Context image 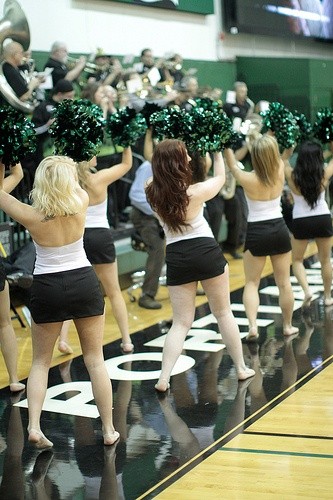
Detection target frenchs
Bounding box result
[0,0,41,113]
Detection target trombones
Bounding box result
[117,79,210,97]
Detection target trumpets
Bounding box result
[67,57,107,75]
[151,59,183,74]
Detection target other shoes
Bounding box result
[222,243,240,259]
[138,295,160,309]
[5,272,33,288]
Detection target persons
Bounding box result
[0,39,333,447]
[145,138,255,391]
[0,308,333,500]
